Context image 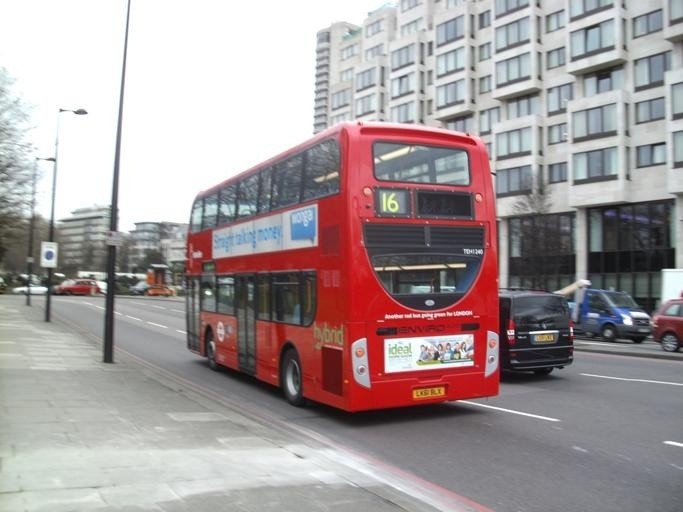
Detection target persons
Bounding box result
[420,342,467,362]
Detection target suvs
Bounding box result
[498,286,575,377]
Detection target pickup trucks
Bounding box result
[562,288,652,342]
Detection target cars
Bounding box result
[138,284,173,296]
[652,299,683,352]
[12,277,101,296]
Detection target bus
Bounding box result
[183,120,503,413]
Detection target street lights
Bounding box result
[44,107,88,323]
[24,156,56,306]
